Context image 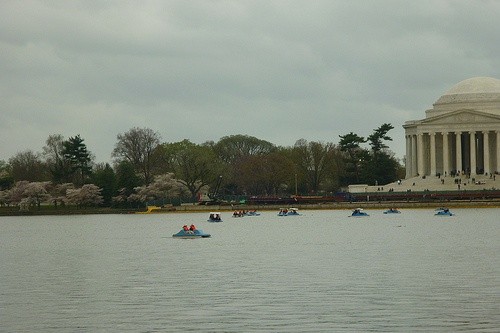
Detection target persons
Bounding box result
[254,209,256,212]
[239,210,246,215]
[183,225,189,231]
[281,209,288,213]
[424,189,429,191]
[398,179,401,185]
[378,187,383,190]
[215,214,220,220]
[485,171,498,181]
[444,208,449,213]
[389,188,393,192]
[210,214,214,219]
[438,169,480,190]
[352,210,357,215]
[234,210,237,215]
[190,224,196,231]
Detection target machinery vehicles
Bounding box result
[198,175,223,205]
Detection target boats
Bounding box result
[232,211,261,218]
[172,229,211,238]
[352,209,367,216]
[383,209,401,214]
[208,212,223,222]
[277,208,299,216]
[435,207,452,216]
